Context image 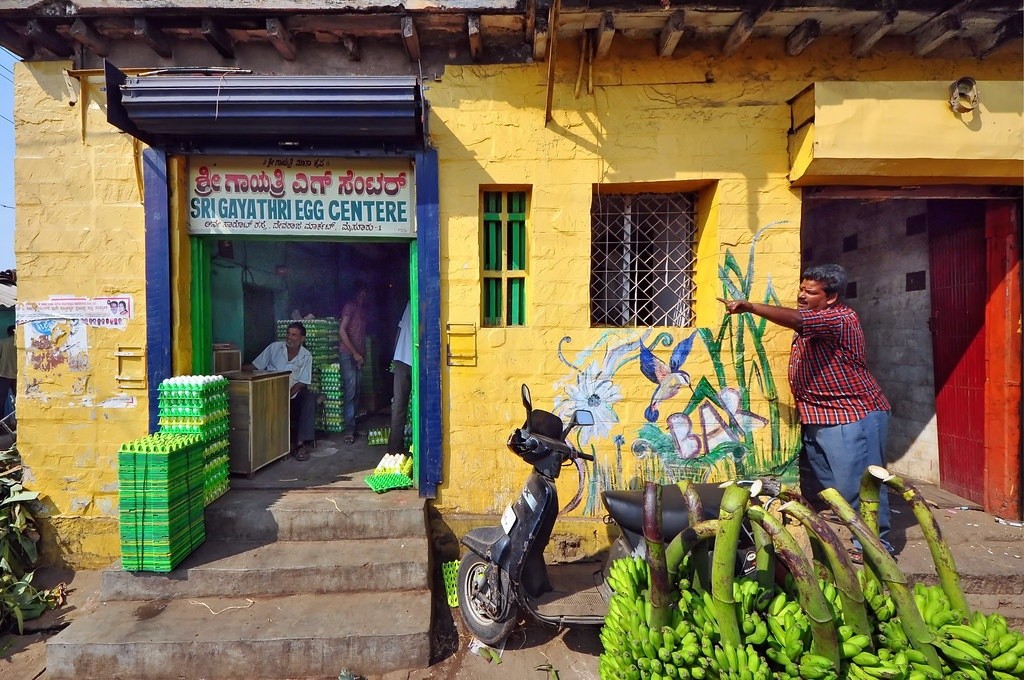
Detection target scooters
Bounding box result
[453,382,793,648]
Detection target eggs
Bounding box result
[162,374,227,501]
[369,431,381,436]
[377,452,404,470]
[277,319,345,431]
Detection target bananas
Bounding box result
[600,552,1024,680]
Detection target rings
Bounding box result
[733,302,735,305]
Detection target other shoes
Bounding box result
[818,510,845,525]
[343,431,355,445]
[296,447,309,461]
[847,548,865,564]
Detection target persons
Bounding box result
[338,280,370,445]
[716,264,896,564]
[110,301,128,315]
[387,300,411,457]
[0,325,16,427]
[242,322,317,461]
